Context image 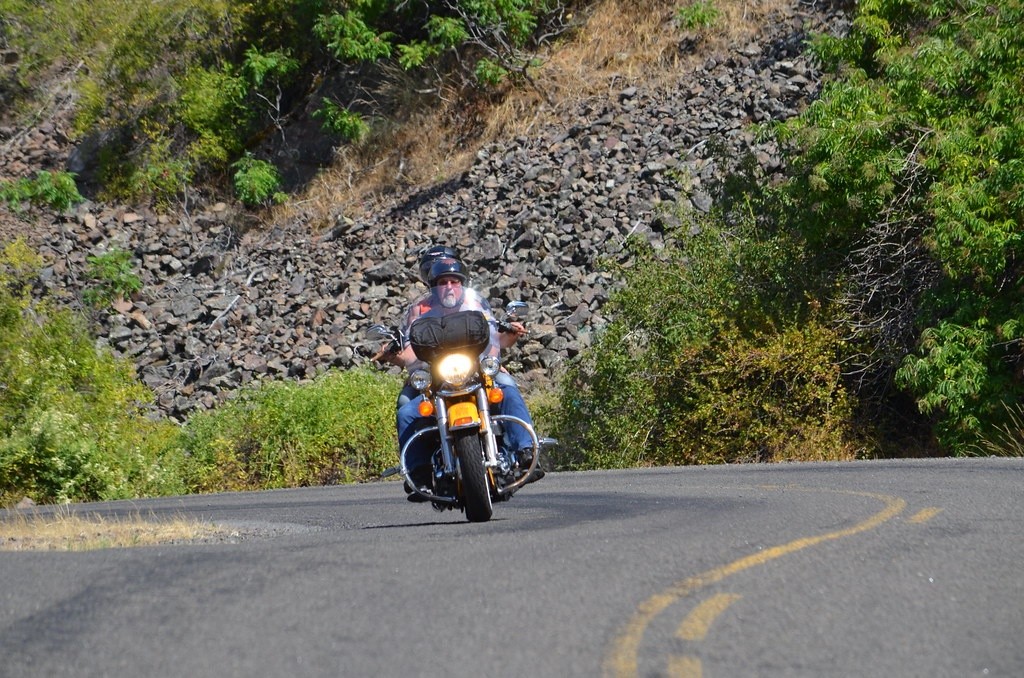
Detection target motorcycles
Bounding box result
[366,300,560,522]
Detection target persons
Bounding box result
[369,244,545,503]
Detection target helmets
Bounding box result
[427,258,470,287]
[419,246,462,287]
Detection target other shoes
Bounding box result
[411,475,427,489]
[519,450,545,481]
